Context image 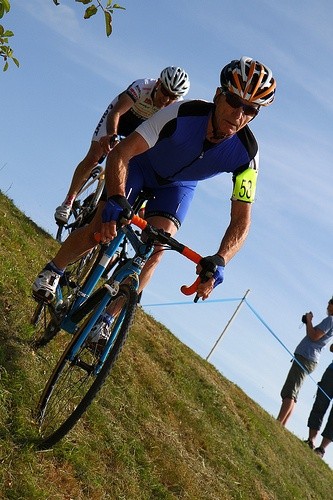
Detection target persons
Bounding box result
[276,298,333,427]
[30,57,277,349]
[54,66,191,223]
[304,343,333,458]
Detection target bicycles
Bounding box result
[54,133,121,283]
[29,188,221,451]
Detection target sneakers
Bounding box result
[89,323,111,350]
[54,205,70,223]
[32,268,61,301]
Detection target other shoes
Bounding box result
[304,439,313,448]
[314,447,325,457]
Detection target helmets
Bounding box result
[160,66,190,96]
[220,56,275,106]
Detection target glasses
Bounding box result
[161,83,178,100]
[222,87,259,116]
